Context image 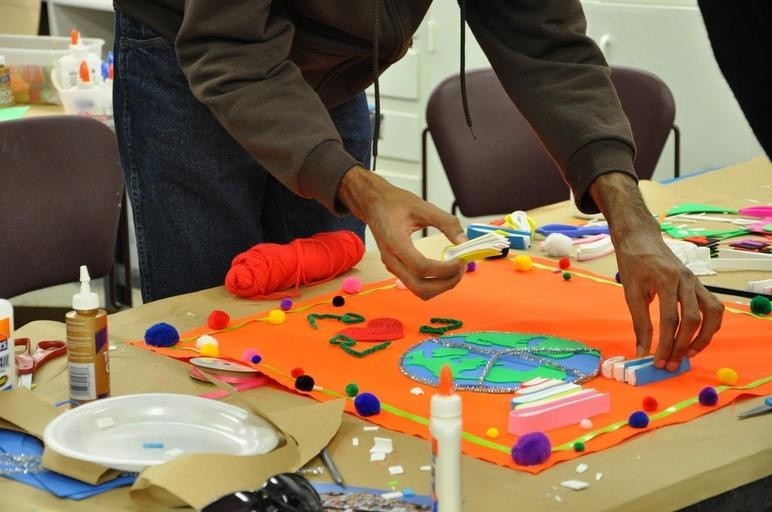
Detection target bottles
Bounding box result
[0,29,114,118]
[58,264,113,403]
[427,365,464,512]
[0,294,15,392]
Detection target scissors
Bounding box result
[14,338,67,388]
[736,396,772,418]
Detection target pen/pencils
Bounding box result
[320,449,344,485]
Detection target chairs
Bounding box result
[422,64,681,219]
[2,117,124,329]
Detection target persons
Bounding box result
[110,1,725,371]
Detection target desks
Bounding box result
[1,149,770,511]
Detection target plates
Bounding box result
[46,391,283,472]
